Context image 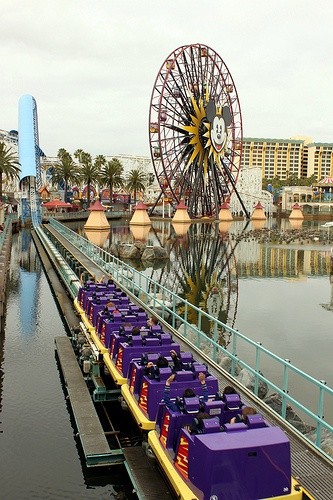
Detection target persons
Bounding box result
[231,209,270,217]
[0,204,18,235]
[43,206,176,216]
[83,272,258,437]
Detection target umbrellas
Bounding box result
[42,196,73,213]
